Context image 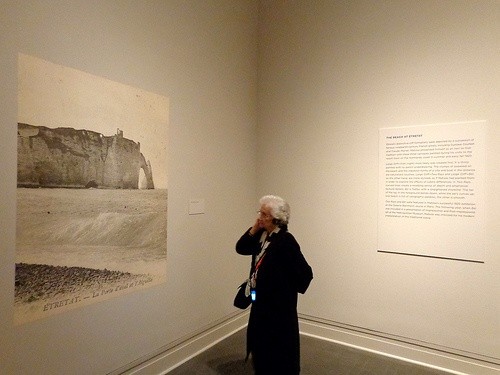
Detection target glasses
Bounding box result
[256,211,271,218]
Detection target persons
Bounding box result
[235,194,313,375]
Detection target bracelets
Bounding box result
[249,231,254,235]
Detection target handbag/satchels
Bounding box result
[233,282,252,309]
